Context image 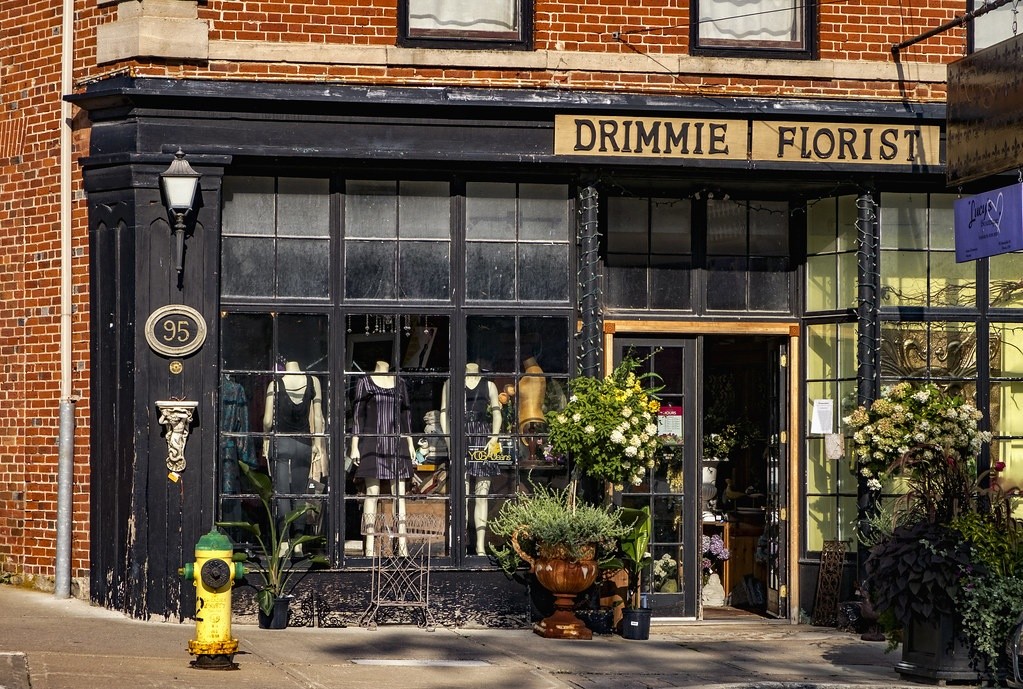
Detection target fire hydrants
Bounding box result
[177,525,250,671]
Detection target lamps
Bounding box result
[158,146,203,275]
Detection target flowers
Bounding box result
[654,553,677,587]
[702,534,730,585]
[543,342,670,488]
[666,425,739,529]
[841,384,1023,689]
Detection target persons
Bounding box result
[350,361,417,560]
[516,358,548,443]
[439,363,506,558]
[219,373,256,515]
[263,360,323,558]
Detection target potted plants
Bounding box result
[213,459,332,631]
[486,486,641,640]
[599,503,651,639]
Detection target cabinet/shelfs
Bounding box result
[675,519,730,610]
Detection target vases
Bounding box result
[660,579,676,592]
[900,593,979,681]
[702,457,731,521]
